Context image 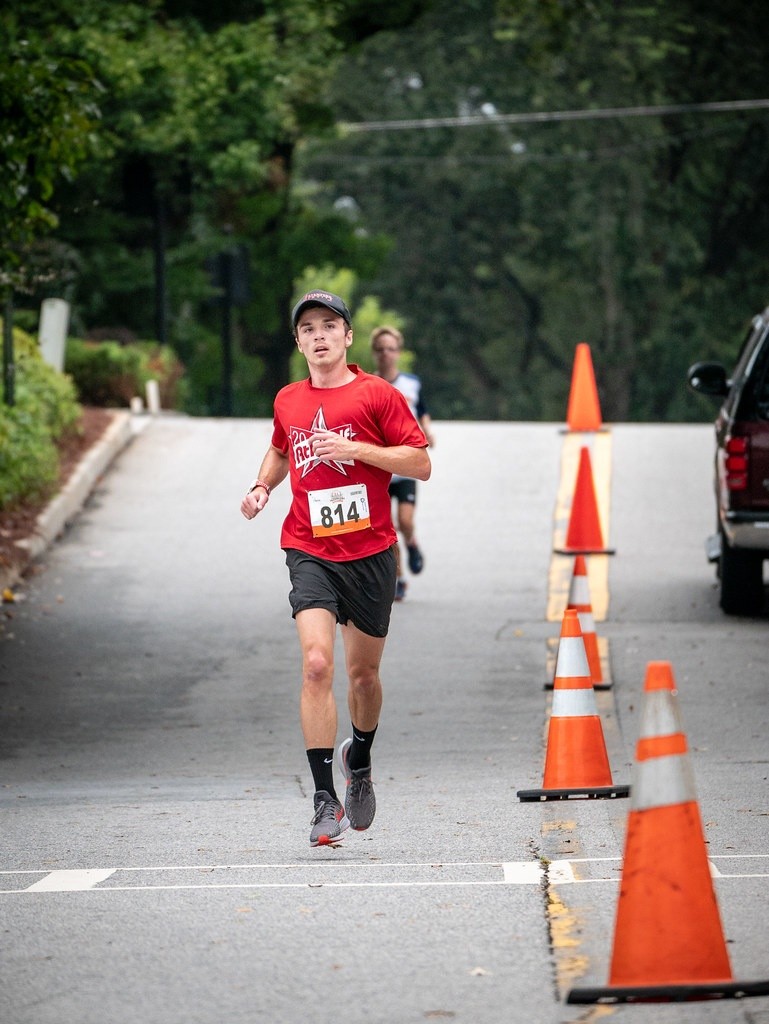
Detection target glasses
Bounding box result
[375,347,402,356]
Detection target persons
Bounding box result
[366,325,436,601]
[241,289,433,847]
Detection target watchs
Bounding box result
[246,480,270,497]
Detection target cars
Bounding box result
[684,307,769,619]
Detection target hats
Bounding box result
[291,289,351,326]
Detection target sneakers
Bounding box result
[337,738,377,832]
[407,537,424,576]
[392,575,408,601]
[307,791,351,848]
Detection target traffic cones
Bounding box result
[558,343,611,435]
[544,554,611,694]
[516,606,632,803]
[550,446,616,555]
[563,661,769,1005]
